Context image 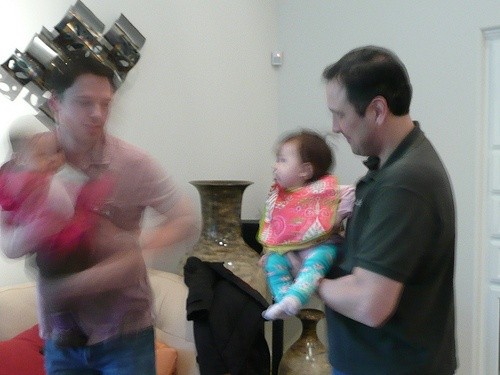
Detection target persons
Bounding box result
[0,114,133,279]
[255,129,357,323]
[0,57,203,375]
[318,44,460,375]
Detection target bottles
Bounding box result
[179,180,268,299]
[279,308,334,375]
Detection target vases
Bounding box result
[177,180,273,375]
[277,308,334,375]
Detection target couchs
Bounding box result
[0,269,271,375]
